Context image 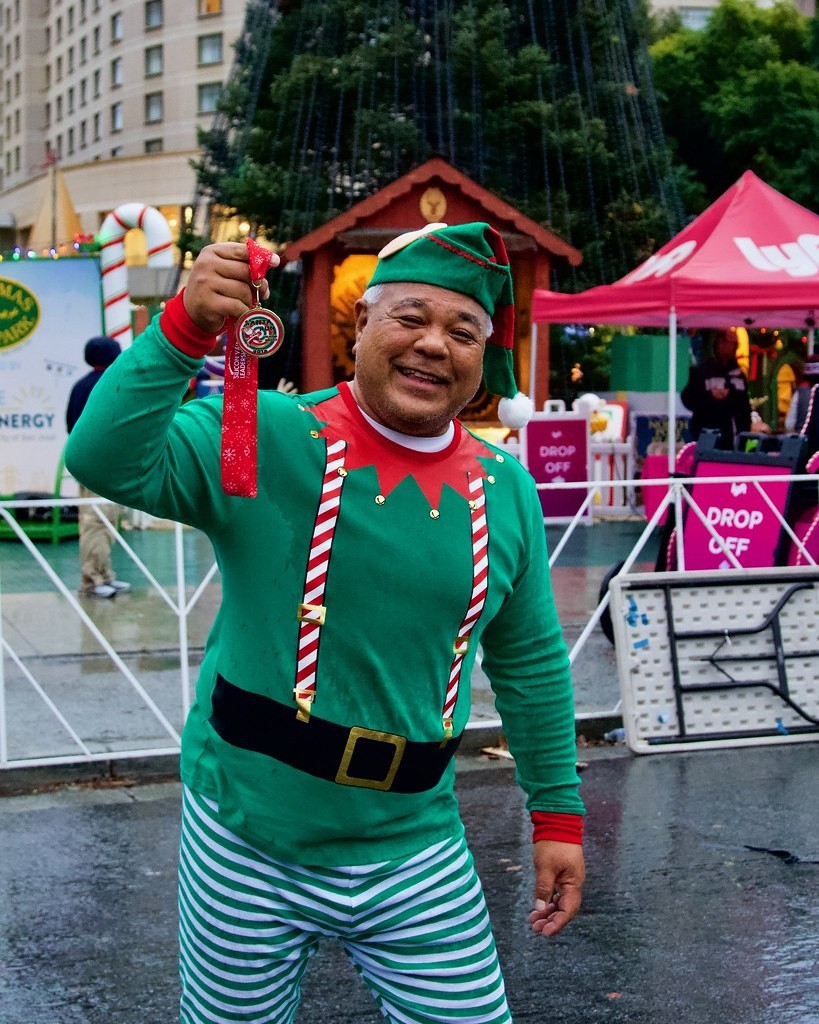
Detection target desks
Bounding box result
[608,565,819,750]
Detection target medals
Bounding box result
[234,307,285,358]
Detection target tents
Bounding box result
[529,171,819,480]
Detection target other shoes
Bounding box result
[105,580,131,593]
[77,584,116,598]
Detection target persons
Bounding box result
[64,221,587,1023]
[785,354,819,472]
[67,337,132,599]
[680,327,752,451]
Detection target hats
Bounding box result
[85,335,121,366]
[365,221,536,431]
